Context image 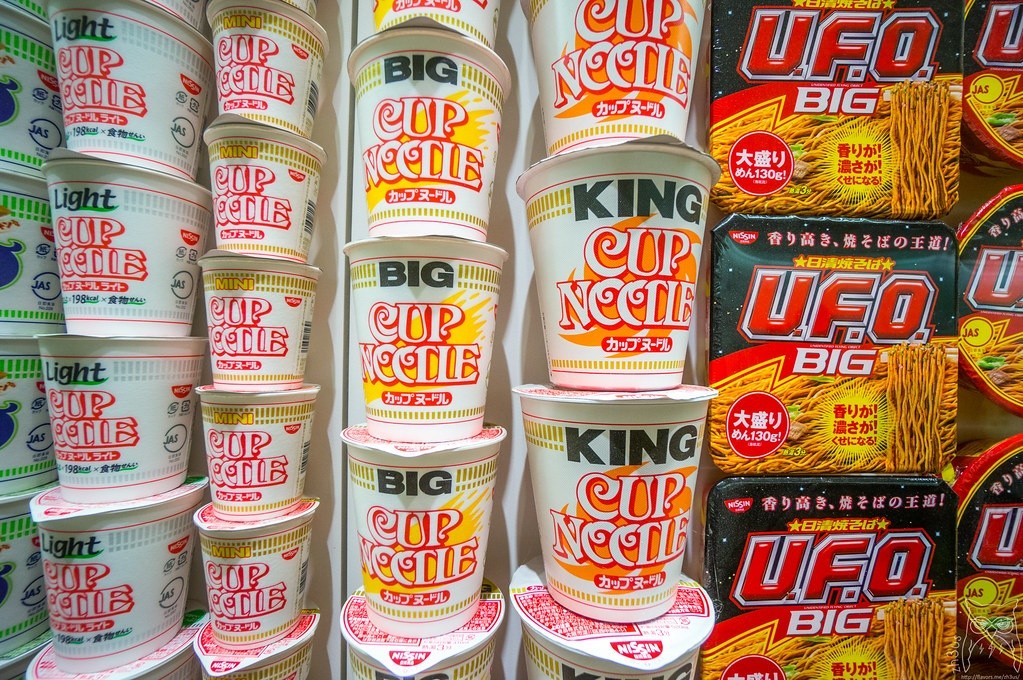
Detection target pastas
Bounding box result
[709,75,1023,680]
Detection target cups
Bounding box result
[2,0,723,678]
